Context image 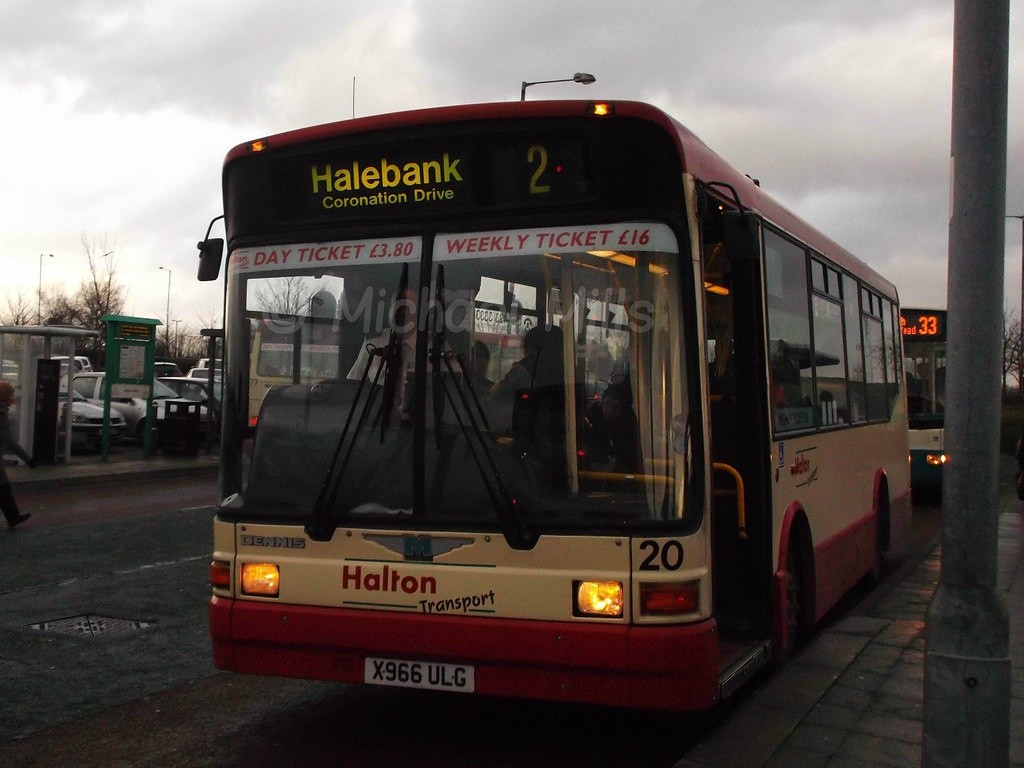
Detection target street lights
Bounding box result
[38,252,56,326]
[159,265,173,353]
[518,69,598,103]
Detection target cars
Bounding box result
[198,359,225,372]
[3,365,20,380]
[58,372,215,443]
[50,355,93,395]
[190,368,226,402]
[7,379,127,451]
[153,362,183,378]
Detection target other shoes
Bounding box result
[9,513,31,527]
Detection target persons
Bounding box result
[1013,432,1024,501]
[466,338,494,395]
[345,278,465,402]
[577,378,640,474]
[0,382,40,530]
[494,323,567,449]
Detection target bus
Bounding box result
[196,100,916,721]
[246,316,617,442]
[901,305,945,492]
